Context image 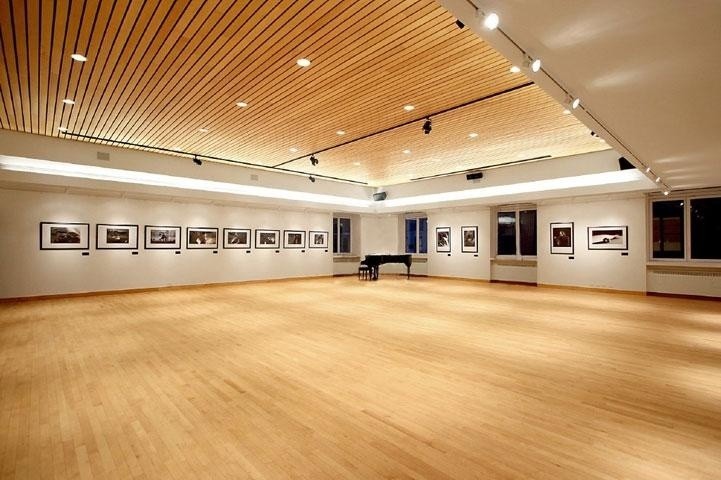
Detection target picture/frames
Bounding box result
[588,226,629,250]
[550,222,575,254]
[436,227,451,252]
[461,225,479,253]
[38,216,330,254]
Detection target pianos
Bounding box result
[361,255,411,279]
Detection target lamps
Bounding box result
[310,114,434,167]
[479,12,583,114]
[193,153,321,182]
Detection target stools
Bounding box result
[359,266,373,281]
